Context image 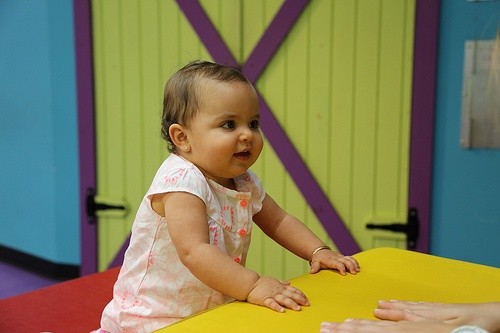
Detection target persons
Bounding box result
[94,60,360,333]
[320,299,500,333]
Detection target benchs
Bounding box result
[0,265,122,333]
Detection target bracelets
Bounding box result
[309,246,331,266]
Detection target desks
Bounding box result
[154,246,500,333]
